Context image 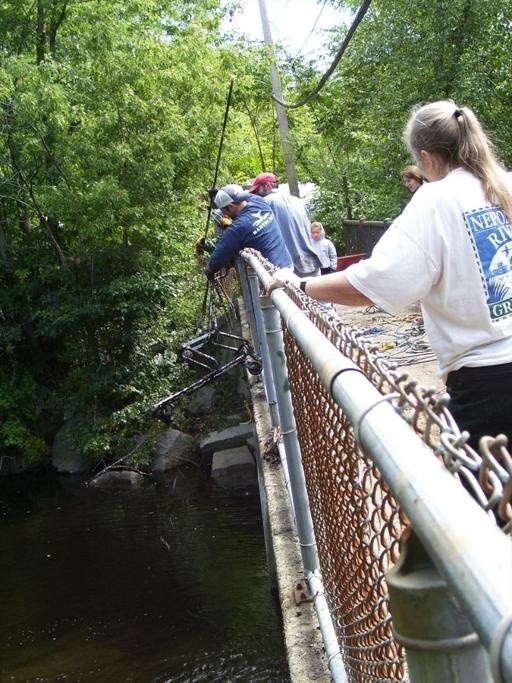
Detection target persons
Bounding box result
[399,164,433,348]
[195,171,337,283]
[265,100,512,499]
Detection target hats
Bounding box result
[250,172,278,192]
[214,184,249,209]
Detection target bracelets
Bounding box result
[300,277,308,292]
